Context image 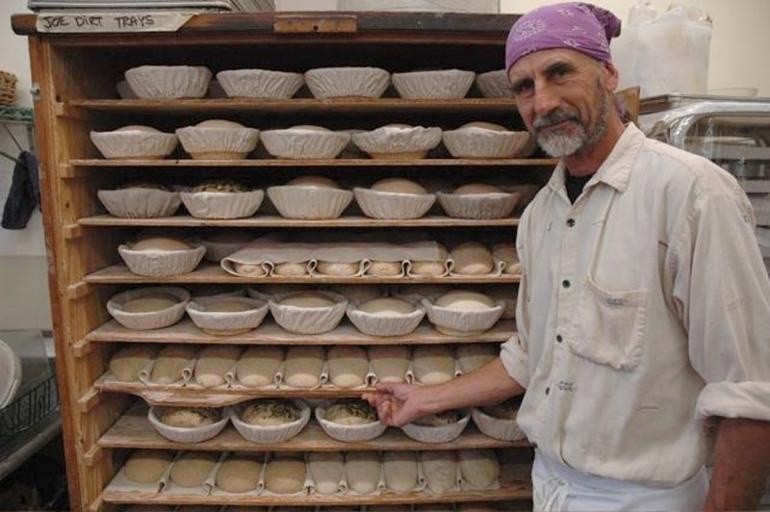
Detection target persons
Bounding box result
[362,2,769,512]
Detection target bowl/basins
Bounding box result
[87,123,535,160]
[95,182,537,220]
[106,287,517,337]
[115,237,251,277]
[114,62,515,100]
[144,396,528,445]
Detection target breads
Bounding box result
[110,119,514,198]
[158,399,520,425]
[124,450,500,497]
[109,343,504,390]
[128,234,520,276]
[104,283,495,312]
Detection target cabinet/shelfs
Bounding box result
[25,34,642,512]
